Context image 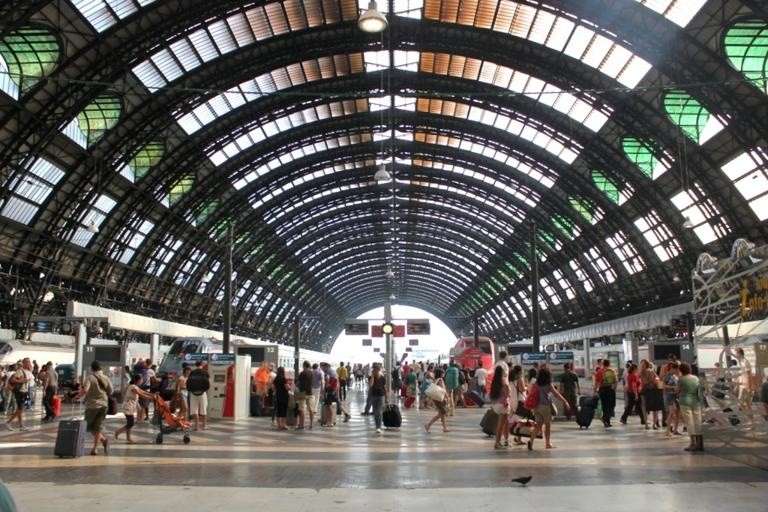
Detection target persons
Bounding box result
[560,362,581,419]
[353,363,370,385]
[361,359,435,433]
[592,357,618,428]
[424,357,489,433]
[62,378,80,402]
[72,360,114,455]
[0,357,58,430]
[0,476,17,512]
[673,362,704,451]
[115,357,211,443]
[488,350,571,451]
[620,347,755,436]
[250,360,351,431]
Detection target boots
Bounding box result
[685,436,704,451]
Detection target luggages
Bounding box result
[54,399,87,458]
[382,400,403,427]
[400,383,416,408]
[320,388,337,427]
[286,390,299,426]
[466,388,486,407]
[575,393,599,428]
[480,409,499,437]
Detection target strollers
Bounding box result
[151,390,194,444]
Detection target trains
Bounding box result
[155,336,338,406]
[0,340,169,377]
[429,334,496,396]
[573,346,648,376]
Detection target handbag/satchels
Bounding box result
[602,368,617,384]
[697,384,710,408]
[515,399,535,419]
[655,380,665,389]
[108,395,118,414]
[52,395,61,414]
[509,420,543,438]
[579,396,598,408]
[424,384,447,402]
[548,398,557,415]
[185,373,210,392]
[524,383,539,411]
[8,375,22,393]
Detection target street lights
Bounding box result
[381,322,393,404]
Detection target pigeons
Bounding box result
[512,475,532,487]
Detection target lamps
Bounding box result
[373,94,393,183]
[383,263,398,300]
[356,1,393,36]
